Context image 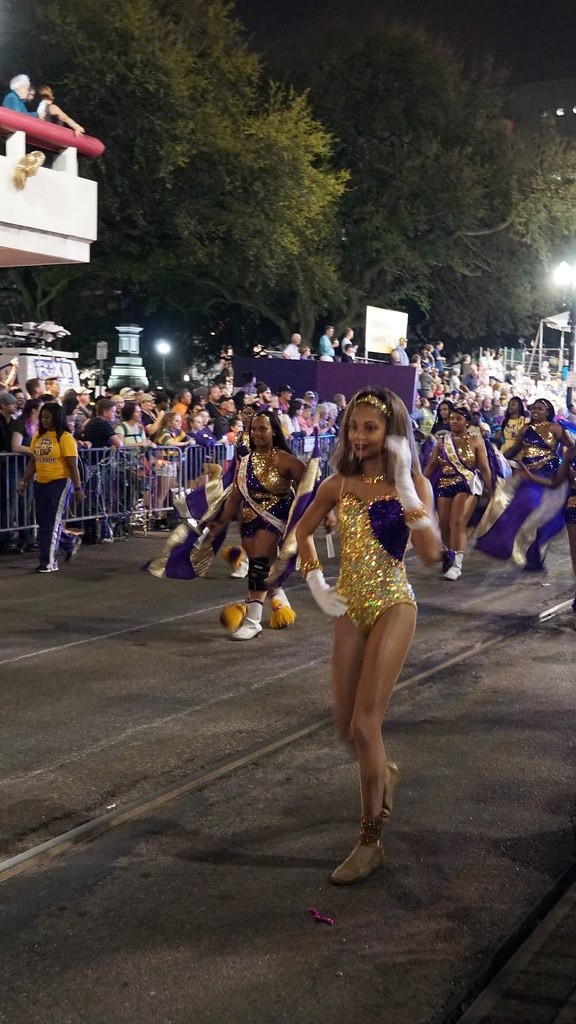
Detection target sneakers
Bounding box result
[36,563,59,572]
[66,536,81,563]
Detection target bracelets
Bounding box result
[19,481,26,485]
[302,560,321,578]
[74,487,82,491]
[408,507,427,521]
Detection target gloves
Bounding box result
[385,434,431,529]
[305,568,350,618]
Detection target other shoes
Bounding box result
[381,762,398,819]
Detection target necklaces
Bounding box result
[360,474,385,484]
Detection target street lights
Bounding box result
[156,343,170,387]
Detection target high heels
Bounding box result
[331,842,385,885]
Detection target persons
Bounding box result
[339,328,357,363]
[3,74,85,165]
[0,378,349,552]
[319,326,337,362]
[207,404,337,638]
[409,342,576,613]
[390,338,409,366]
[17,403,84,572]
[298,346,311,360]
[297,386,439,885]
[283,333,301,359]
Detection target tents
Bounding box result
[527,310,572,372]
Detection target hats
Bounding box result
[278,384,295,393]
[120,387,136,399]
[305,391,316,398]
[256,385,269,393]
[0,393,18,405]
[400,338,409,341]
[138,394,156,402]
[428,398,440,403]
[423,363,430,368]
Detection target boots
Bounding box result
[441,549,464,581]
[231,553,249,579]
[153,516,171,532]
[232,597,264,642]
[269,586,291,627]
